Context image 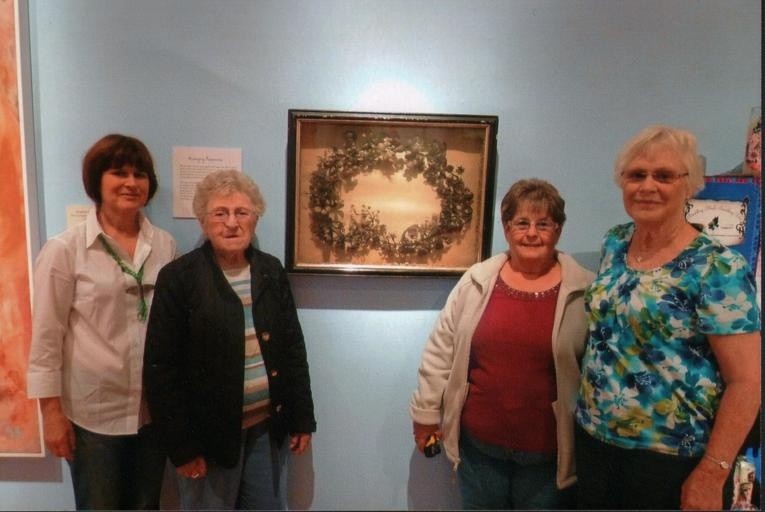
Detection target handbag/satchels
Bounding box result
[703,454,732,470]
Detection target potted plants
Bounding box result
[2,1,47,460]
[286,105,498,277]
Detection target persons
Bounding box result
[26,133,180,511]
[140,168,317,509]
[580,120,762,512]
[414,177,601,509]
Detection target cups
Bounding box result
[512,219,559,230]
[209,210,253,223]
[624,171,689,182]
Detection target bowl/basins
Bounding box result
[628,222,689,264]
[508,253,553,276]
